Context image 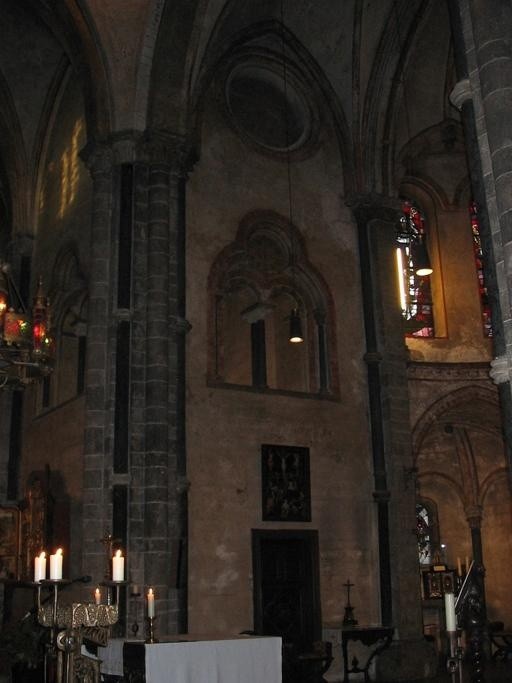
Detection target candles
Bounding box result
[34,549,49,584]
[111,547,127,582]
[50,547,64,578]
[146,590,157,618]
[444,591,456,631]
[94,588,103,604]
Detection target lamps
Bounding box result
[282,0,308,346]
[0,252,53,391]
[396,0,433,283]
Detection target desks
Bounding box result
[80,632,283,681]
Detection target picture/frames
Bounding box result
[260,441,312,522]
